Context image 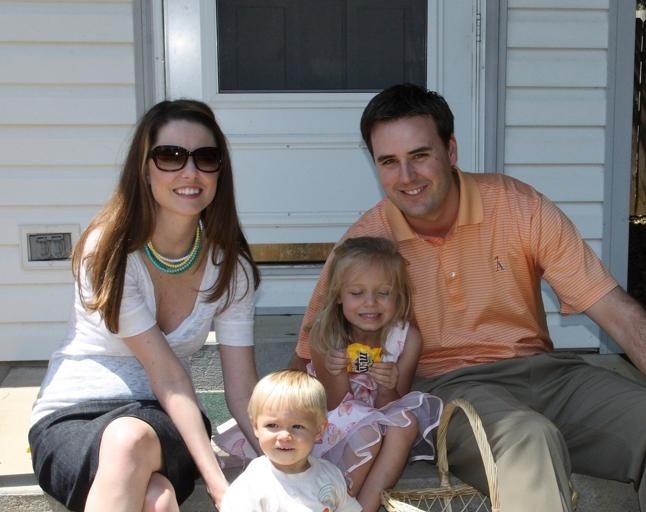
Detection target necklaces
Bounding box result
[142,225,204,278]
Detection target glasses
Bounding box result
[147,145,223,174]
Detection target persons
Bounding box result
[25,98,358,512]
[276,78,646,512]
[309,234,422,511]
[217,370,364,511]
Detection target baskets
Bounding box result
[379,393,580,512]
